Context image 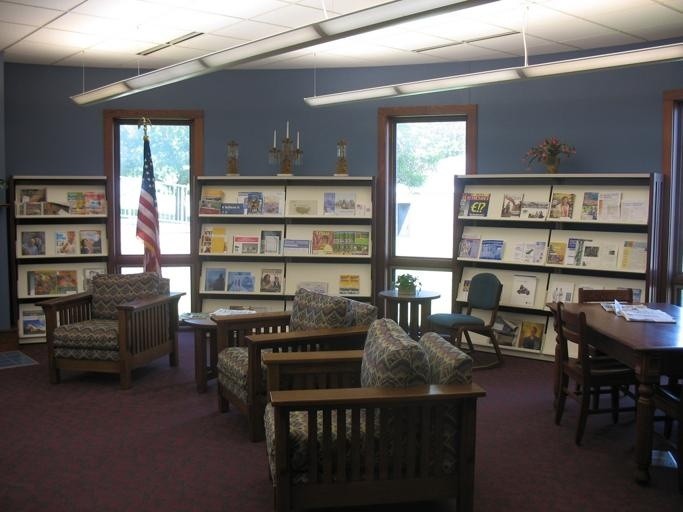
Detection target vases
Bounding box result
[545,158,561,174]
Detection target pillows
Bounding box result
[89,274,158,316]
[290,288,349,329]
[360,319,427,386]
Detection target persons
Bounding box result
[321,235,333,252]
[60,232,76,253]
[261,274,272,292]
[273,277,280,291]
[81,239,99,253]
[22,238,39,255]
[523,326,542,348]
[557,196,569,217]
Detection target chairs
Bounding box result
[427,273,502,366]
[553,302,638,448]
[574,287,633,411]
[652,384,683,451]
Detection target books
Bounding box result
[579,284,604,303]
[298,281,328,297]
[621,199,648,224]
[21,231,46,256]
[289,200,318,216]
[355,202,364,218]
[57,271,78,294]
[597,193,622,224]
[332,230,369,255]
[22,309,48,338]
[517,320,545,350]
[55,231,79,254]
[618,286,642,302]
[260,230,282,255]
[283,239,311,255]
[339,274,360,295]
[581,241,626,270]
[68,191,106,214]
[612,300,676,322]
[27,270,35,296]
[510,275,536,307]
[457,238,480,258]
[205,268,226,291]
[209,308,257,317]
[458,192,491,216]
[237,191,263,214]
[501,192,524,217]
[312,231,333,255]
[16,187,70,216]
[80,230,102,254]
[365,203,372,217]
[260,268,283,293]
[479,240,504,260]
[489,315,524,347]
[227,271,255,292]
[545,282,575,310]
[520,201,549,220]
[199,190,224,214]
[84,268,107,290]
[264,191,286,216]
[458,279,472,301]
[566,238,593,265]
[135,139,162,278]
[199,226,225,253]
[547,242,566,264]
[222,203,244,214]
[580,192,600,220]
[523,241,546,264]
[35,270,57,295]
[324,192,356,218]
[548,191,576,220]
[233,236,260,254]
[618,239,648,273]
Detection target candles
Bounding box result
[285,120,291,140]
[273,129,277,149]
[296,130,300,151]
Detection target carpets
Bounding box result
[1,350,40,370]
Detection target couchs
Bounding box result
[263,330,488,512]
[211,300,376,442]
[35,275,186,389]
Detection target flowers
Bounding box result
[521,136,578,171]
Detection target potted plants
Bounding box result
[396,274,416,295]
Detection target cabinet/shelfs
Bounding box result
[453,173,547,361]
[543,170,663,362]
[285,177,376,310]
[196,175,285,310]
[7,175,110,344]
